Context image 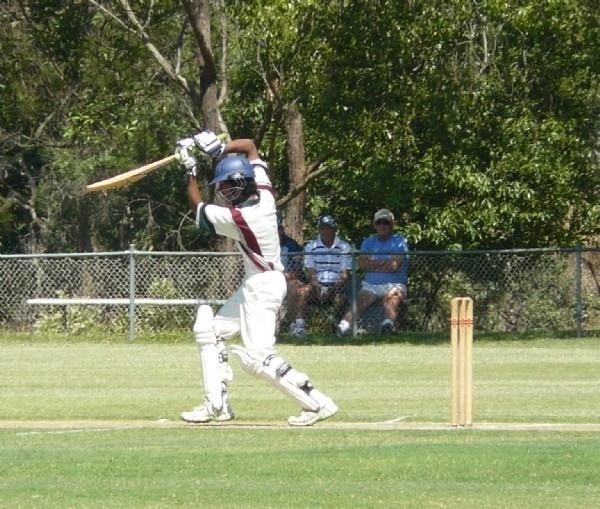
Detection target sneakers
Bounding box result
[289,322,305,339]
[379,319,396,334]
[287,396,339,427]
[337,322,352,338]
[181,396,235,424]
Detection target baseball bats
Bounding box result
[84,131,229,192]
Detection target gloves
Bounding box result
[193,131,226,159]
[175,138,198,177]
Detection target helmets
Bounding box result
[208,155,256,186]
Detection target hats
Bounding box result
[374,208,395,221]
[317,214,337,227]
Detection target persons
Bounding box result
[331,208,412,339]
[174,128,340,427]
[274,219,303,338]
[291,214,353,339]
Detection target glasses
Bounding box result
[375,220,389,225]
